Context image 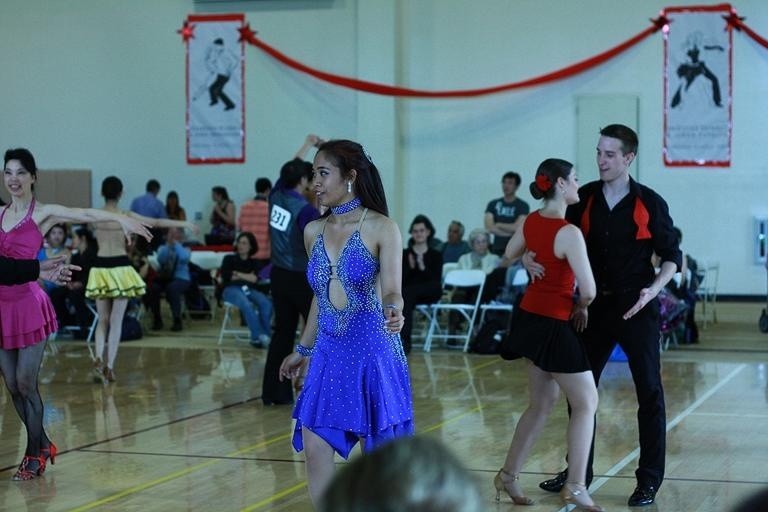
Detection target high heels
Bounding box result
[494,469,537,505]
[91,358,116,384]
[560,480,605,512]
[12,442,57,481]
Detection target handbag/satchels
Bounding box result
[92,315,142,342]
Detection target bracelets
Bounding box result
[295,344,316,357]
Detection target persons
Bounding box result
[261,135,319,405]
[671,32,724,107]
[494,158,604,511]
[206,171,707,349]
[278,139,415,512]
[38,176,198,381]
[0,147,155,481]
[208,38,238,111]
[538,124,682,507]
[318,435,484,512]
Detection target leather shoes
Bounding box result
[264,395,293,405]
[539,472,590,492]
[628,485,657,506]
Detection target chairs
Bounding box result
[47,246,275,348]
